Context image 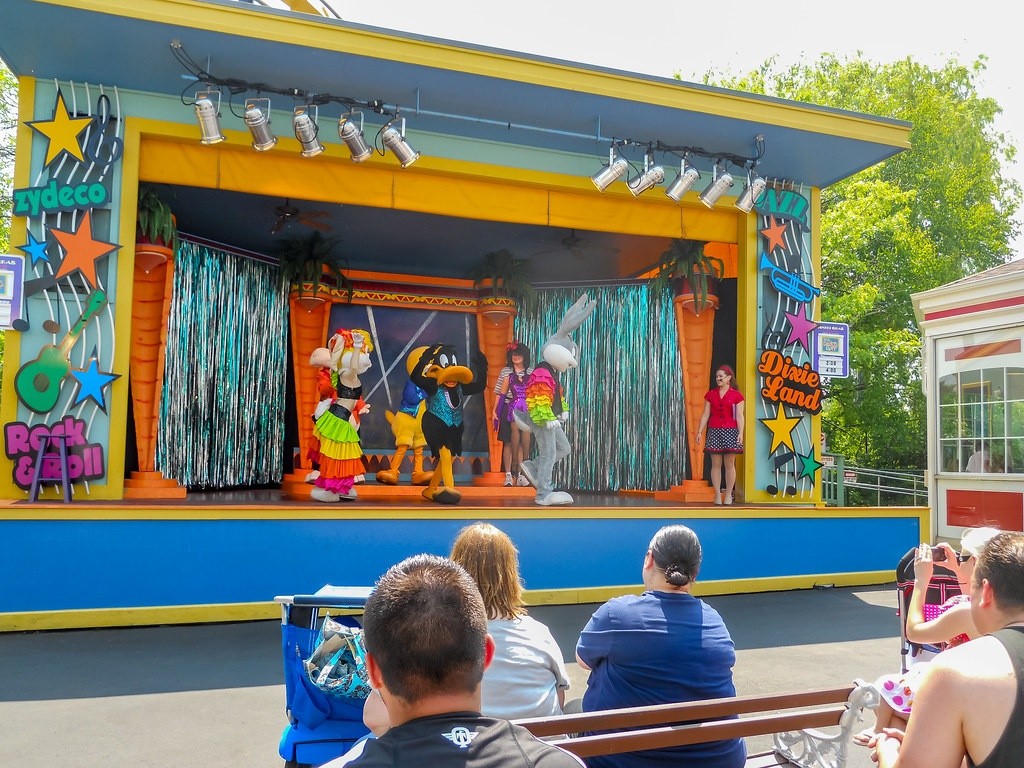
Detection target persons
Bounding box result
[321,554,587,768]
[566,523,747,768]
[695,366,745,505]
[363,522,571,740]
[492,343,533,486]
[852,526,1002,747]
[965,441,991,473]
[868,530,1024,768]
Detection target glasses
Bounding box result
[956,554,971,567]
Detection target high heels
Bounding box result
[714,495,722,505]
[724,494,733,506]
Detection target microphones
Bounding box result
[720,376,727,381]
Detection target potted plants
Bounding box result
[136,180,179,261]
[272,230,354,305]
[475,247,539,316]
[647,237,726,317]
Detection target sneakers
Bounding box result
[503,475,513,487]
[517,476,529,486]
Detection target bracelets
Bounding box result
[698,432,702,433]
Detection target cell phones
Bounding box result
[930,547,946,561]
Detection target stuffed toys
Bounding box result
[310,328,373,502]
[375,346,435,485]
[514,294,597,505]
[411,345,489,503]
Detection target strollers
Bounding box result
[896,547,964,674]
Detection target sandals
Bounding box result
[852,733,874,747]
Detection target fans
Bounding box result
[531,229,621,261]
[230,197,332,236]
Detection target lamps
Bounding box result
[590,147,628,193]
[625,154,665,199]
[733,169,766,214]
[338,107,373,162]
[292,104,325,158]
[195,85,225,144]
[244,98,277,151]
[380,104,420,169]
[664,152,701,204]
[698,165,733,209]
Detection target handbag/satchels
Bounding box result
[303,616,371,698]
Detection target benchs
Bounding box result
[508,678,880,768]
